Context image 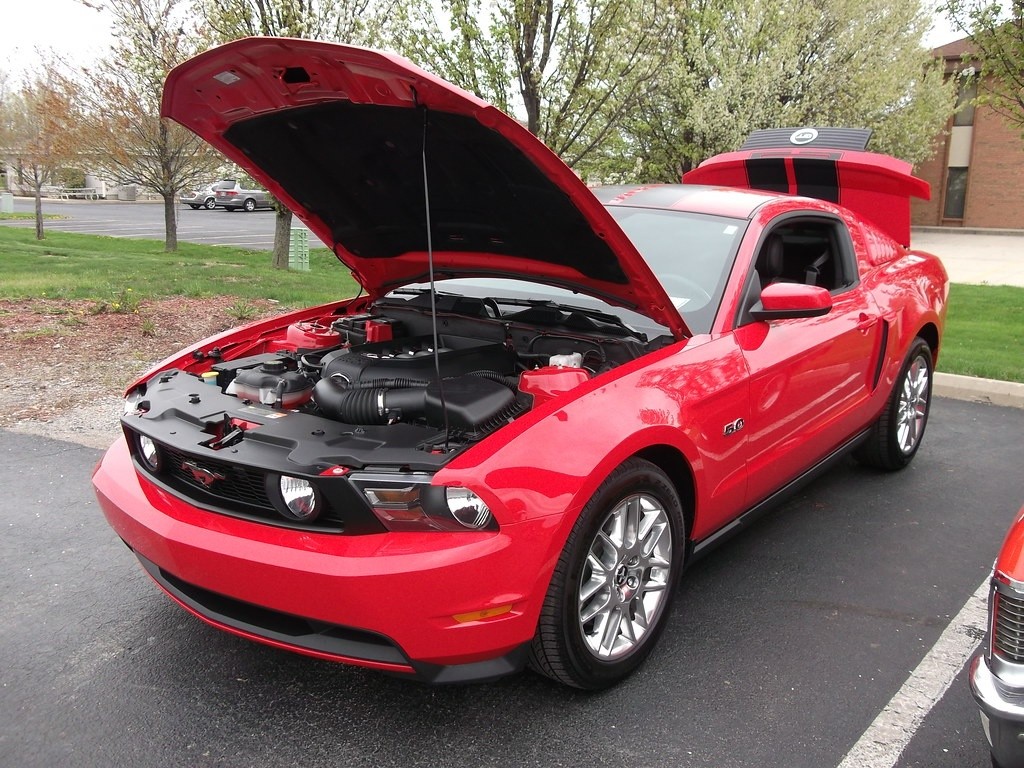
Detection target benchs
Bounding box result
[56,187,103,201]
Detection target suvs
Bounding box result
[214,176,277,213]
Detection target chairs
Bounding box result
[755,233,787,289]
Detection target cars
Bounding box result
[179,183,219,210]
[88,35,947,695]
